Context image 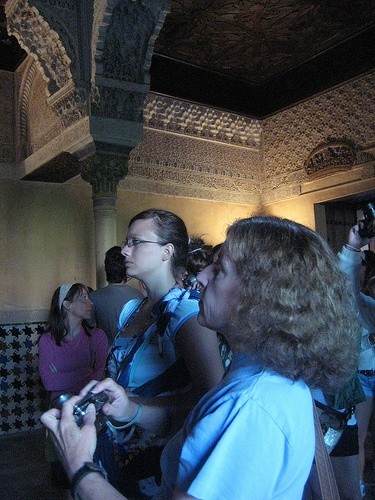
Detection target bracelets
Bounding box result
[70,462,106,500]
[346,243,360,251]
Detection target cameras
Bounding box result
[360,202,375,238]
[54,389,110,428]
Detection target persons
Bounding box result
[302,217,375,500]
[40,216,357,500]
[106,208,224,500]
[39,283,109,479]
[88,246,144,346]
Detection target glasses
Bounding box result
[121,239,167,250]
